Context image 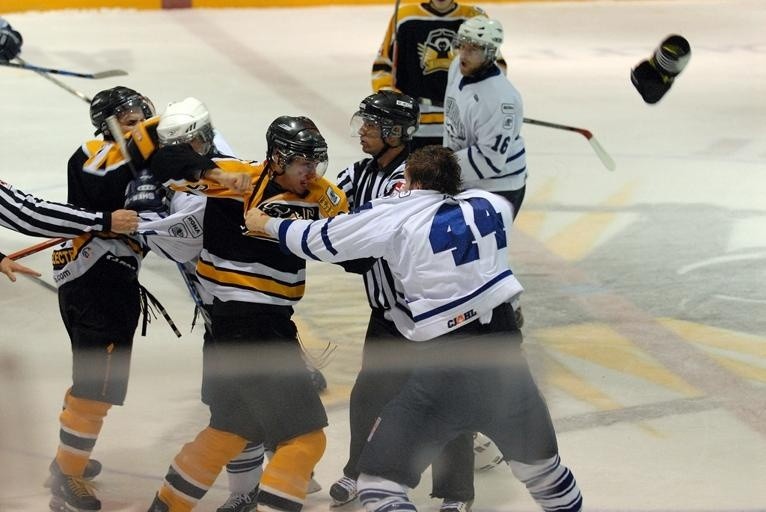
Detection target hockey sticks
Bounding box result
[524,118,616,172]
[1,61,126,78]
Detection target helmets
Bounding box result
[349,90,420,142]
[451,15,504,49]
[89,86,156,141]
[265,114,329,188]
[156,97,215,156]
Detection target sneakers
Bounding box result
[216,483,260,512]
[439,495,475,512]
[82,459,102,478]
[51,476,101,511]
[329,474,357,501]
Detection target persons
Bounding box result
[443,15,528,344]
[43,87,156,512]
[246,149,582,512]
[371,1,508,154]
[123,97,322,511]
[147,115,379,511]
[0,180,138,282]
[0,17,22,63]
[330,91,474,511]
